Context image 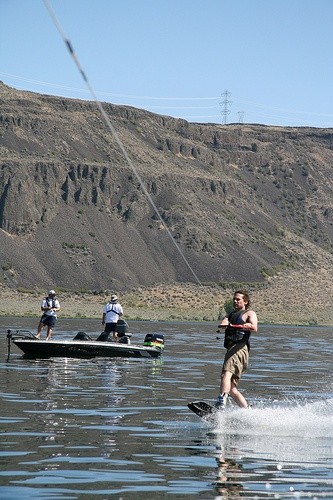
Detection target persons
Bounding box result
[32,290,60,341]
[101,294,122,340]
[213,290,257,413]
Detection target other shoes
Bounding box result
[213,393,228,409]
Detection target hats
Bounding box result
[49,290,55,294]
[111,295,117,300]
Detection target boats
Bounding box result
[7,328,166,358]
